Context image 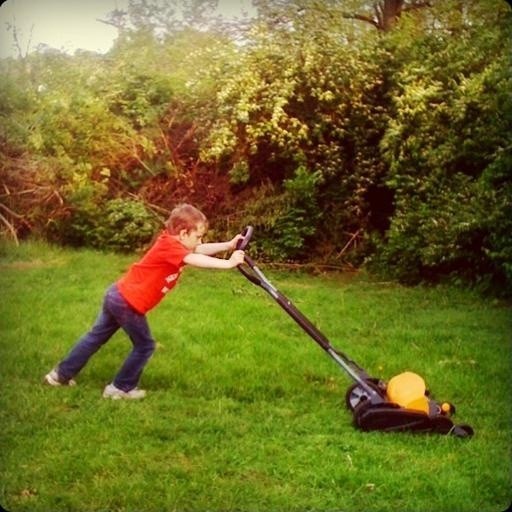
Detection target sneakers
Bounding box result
[45,368,78,387]
[101,383,148,401]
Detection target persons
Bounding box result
[45,203,246,399]
